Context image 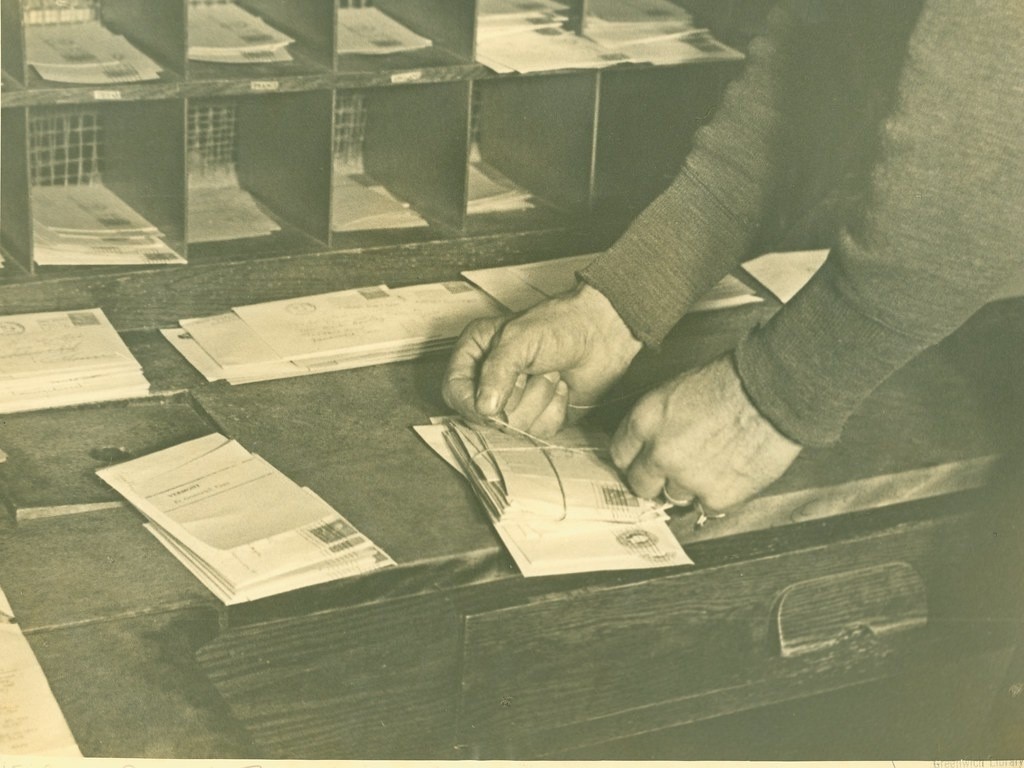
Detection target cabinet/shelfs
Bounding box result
[3,2,1022,761]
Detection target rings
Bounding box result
[695,502,726,525]
[662,487,693,506]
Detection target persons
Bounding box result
[443,0,1023,519]
